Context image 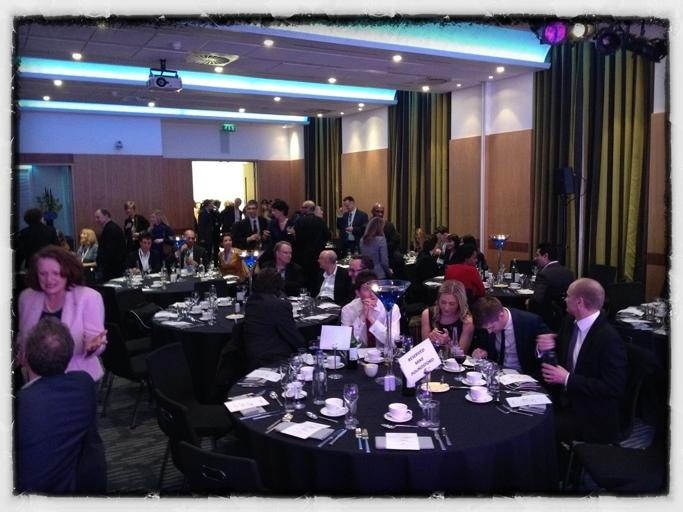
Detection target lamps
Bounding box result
[536,16,589,46]
[594,24,666,64]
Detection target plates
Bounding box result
[461,378,486,386]
[297,374,313,381]
[151,284,163,287]
[188,310,204,314]
[224,313,245,320]
[465,395,492,405]
[364,357,385,363]
[384,411,413,422]
[320,406,349,417]
[442,365,466,374]
[508,287,523,290]
[324,362,345,369]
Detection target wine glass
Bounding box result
[416,385,432,428]
[454,349,468,370]
[342,383,359,429]
[365,279,411,385]
[191,291,200,306]
[480,360,494,377]
[289,352,302,381]
[237,247,264,298]
[489,229,511,273]
[299,288,308,301]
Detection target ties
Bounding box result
[253,220,257,233]
[566,323,579,372]
[348,213,352,227]
[365,318,376,348]
[498,329,505,365]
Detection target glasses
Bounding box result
[301,206,307,209]
[348,267,363,274]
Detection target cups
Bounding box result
[469,386,486,399]
[364,364,378,377]
[367,350,381,361]
[153,280,163,286]
[427,401,440,429]
[324,396,342,411]
[284,384,296,413]
[278,363,290,389]
[170,273,177,283]
[327,356,341,366]
[509,282,520,288]
[444,358,458,368]
[192,305,201,312]
[290,380,303,394]
[387,400,410,417]
[530,275,537,281]
[299,366,316,376]
[466,372,483,384]
[486,369,502,401]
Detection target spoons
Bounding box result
[306,411,339,423]
[378,422,418,430]
[269,390,282,408]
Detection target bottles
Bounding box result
[312,351,328,406]
[208,284,217,310]
[197,257,205,275]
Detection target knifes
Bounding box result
[317,427,347,448]
[431,429,445,451]
[329,430,347,446]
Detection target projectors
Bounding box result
[146,59,182,94]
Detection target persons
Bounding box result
[13,316,107,495]
[14,209,60,286]
[238,226,579,384]
[15,241,107,398]
[537,275,628,497]
[76,197,402,282]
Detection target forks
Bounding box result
[441,426,454,449]
[361,427,371,454]
[505,384,541,391]
[353,427,362,451]
[496,404,543,417]
